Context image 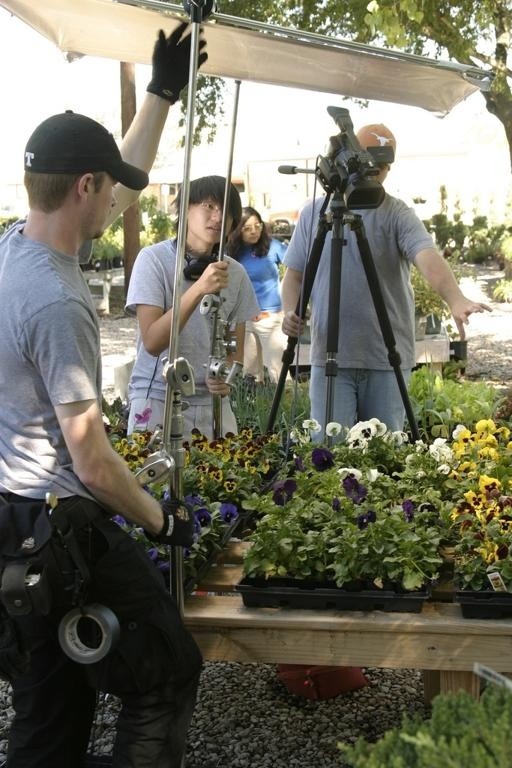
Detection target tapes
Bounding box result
[57,603,120,665]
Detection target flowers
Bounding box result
[105,408,512,592]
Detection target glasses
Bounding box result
[241,222,264,232]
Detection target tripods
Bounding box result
[264,185,422,447]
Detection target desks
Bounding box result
[180,541,512,707]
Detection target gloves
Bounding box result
[144,498,195,548]
[147,22,208,105]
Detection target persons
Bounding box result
[224,206,294,383]
[278,123,493,448]
[125,174,261,440]
[0,13,206,767]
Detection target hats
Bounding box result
[23,110,149,191]
[356,123,396,154]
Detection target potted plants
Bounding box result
[411,252,478,360]
[278,263,311,344]
